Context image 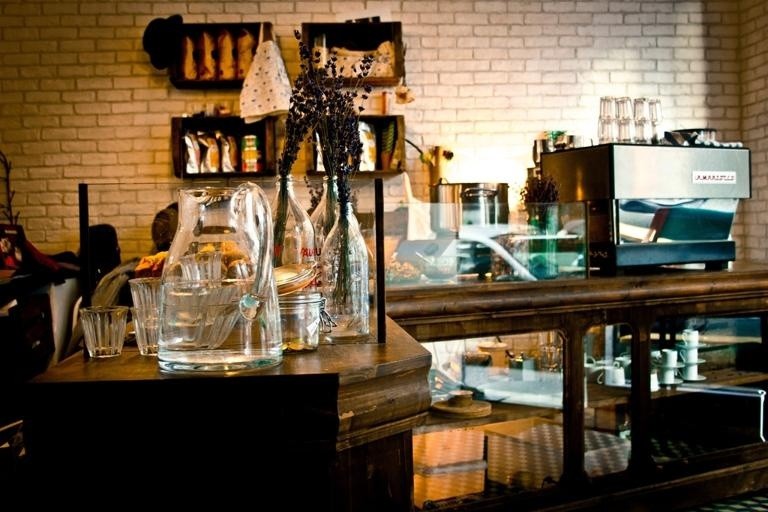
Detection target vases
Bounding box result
[269,174,372,342]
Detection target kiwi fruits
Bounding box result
[165,240,253,279]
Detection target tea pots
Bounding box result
[154,180,285,376]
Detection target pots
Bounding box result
[428,181,511,238]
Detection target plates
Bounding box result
[650,342,711,388]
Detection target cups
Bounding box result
[598,94,664,144]
[581,328,700,385]
[76,274,162,359]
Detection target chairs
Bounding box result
[79,224,126,303]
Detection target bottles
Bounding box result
[268,167,373,354]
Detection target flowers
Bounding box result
[263,27,388,307]
